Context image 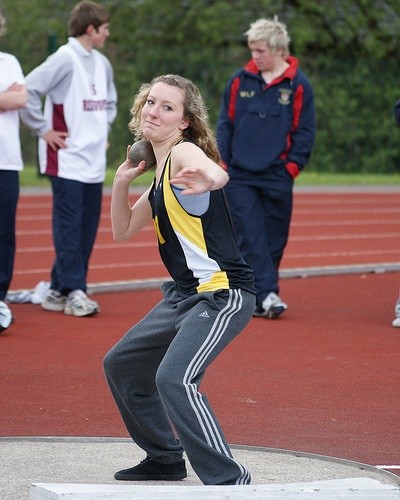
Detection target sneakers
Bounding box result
[65,290,100,316]
[41,288,65,311]
[0,300,13,330]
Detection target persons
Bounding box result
[216,14,315,320]
[18,1,117,316]
[0,13,27,330]
[102,74,256,486]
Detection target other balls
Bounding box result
[128,140,157,170]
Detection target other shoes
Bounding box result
[262,291,288,318]
[115,455,188,480]
